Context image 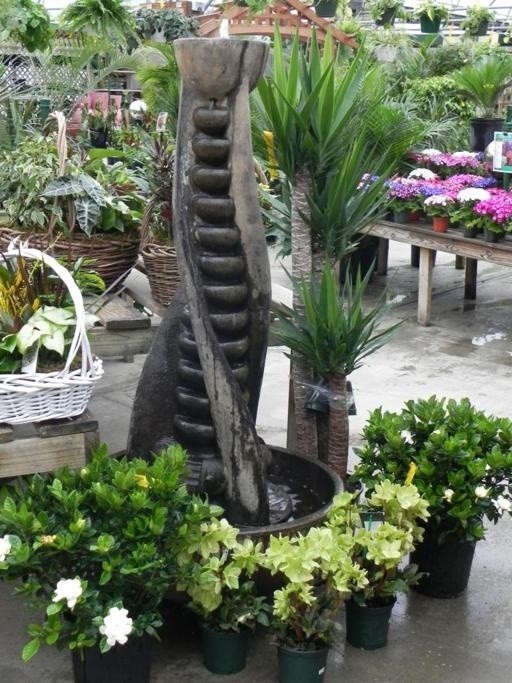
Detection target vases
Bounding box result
[66,617,159,682]
[403,508,481,599]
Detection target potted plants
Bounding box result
[310,0,511,48]
[267,522,370,683]
[171,515,271,675]
[322,482,433,651]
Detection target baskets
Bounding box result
[136,181,184,307]
[0,108,144,295]
[0,248,106,428]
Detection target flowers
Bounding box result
[1,239,107,373]
[355,145,512,244]
[349,392,511,547]
[0,439,217,661]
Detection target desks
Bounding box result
[333,216,511,329]
